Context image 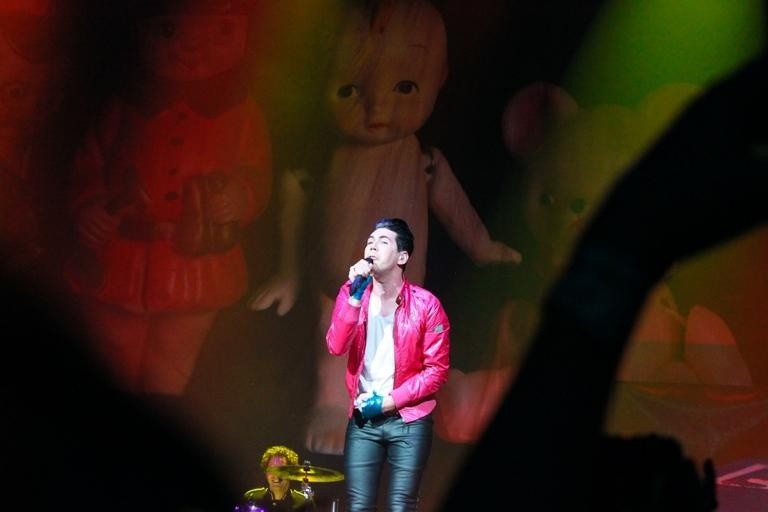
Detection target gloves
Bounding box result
[354,392,383,424]
[348,275,373,300]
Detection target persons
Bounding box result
[249,0,526,456]
[237,442,313,511]
[326,214,453,511]
[66,2,278,400]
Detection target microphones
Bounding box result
[349,257,373,296]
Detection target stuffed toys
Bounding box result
[496,77,755,387]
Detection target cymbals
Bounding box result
[267,464,344,483]
